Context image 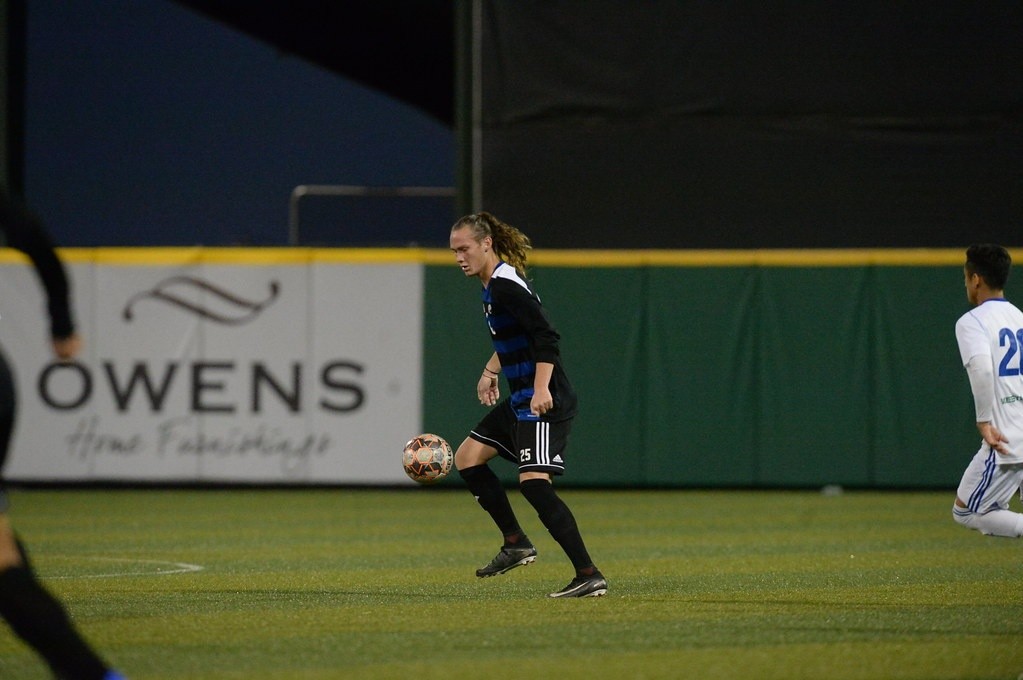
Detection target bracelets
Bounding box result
[485,367,499,375]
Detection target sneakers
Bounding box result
[550,569,607,599]
[476,540,537,578]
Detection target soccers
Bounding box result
[401,433,455,484]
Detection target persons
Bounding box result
[449,212,608,598]
[0,188,121,680]
[953,244,1023,536]
